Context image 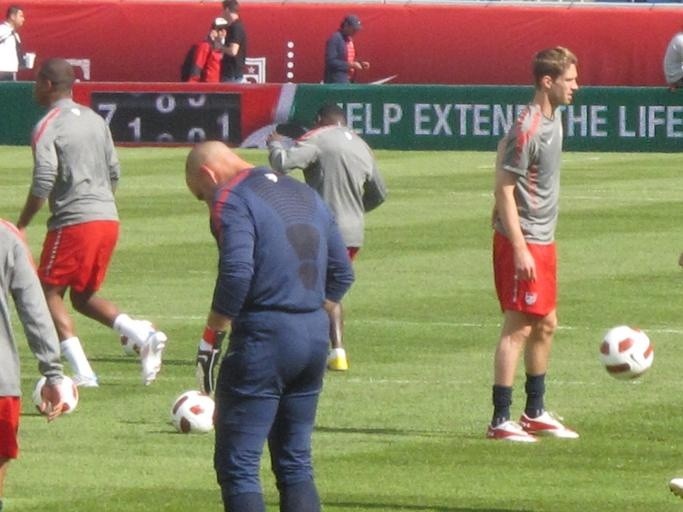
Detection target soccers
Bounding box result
[172,391,214,434]
[35,374,79,416]
[600,326,653,379]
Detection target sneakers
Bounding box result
[137,331,170,388]
[486,421,539,443]
[326,347,349,372]
[519,410,581,439]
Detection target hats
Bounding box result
[212,16,229,29]
[345,15,364,32]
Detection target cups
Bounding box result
[24,52,36,69]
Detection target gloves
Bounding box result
[197,325,228,396]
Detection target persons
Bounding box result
[184,140,356,512]
[0,217,65,512]
[0,5,24,81]
[186,16,228,82]
[663,32,683,88]
[266,105,388,372]
[324,14,370,84]
[486,45,580,443]
[16,57,166,387]
[209,0,248,84]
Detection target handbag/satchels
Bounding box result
[360,176,388,213]
